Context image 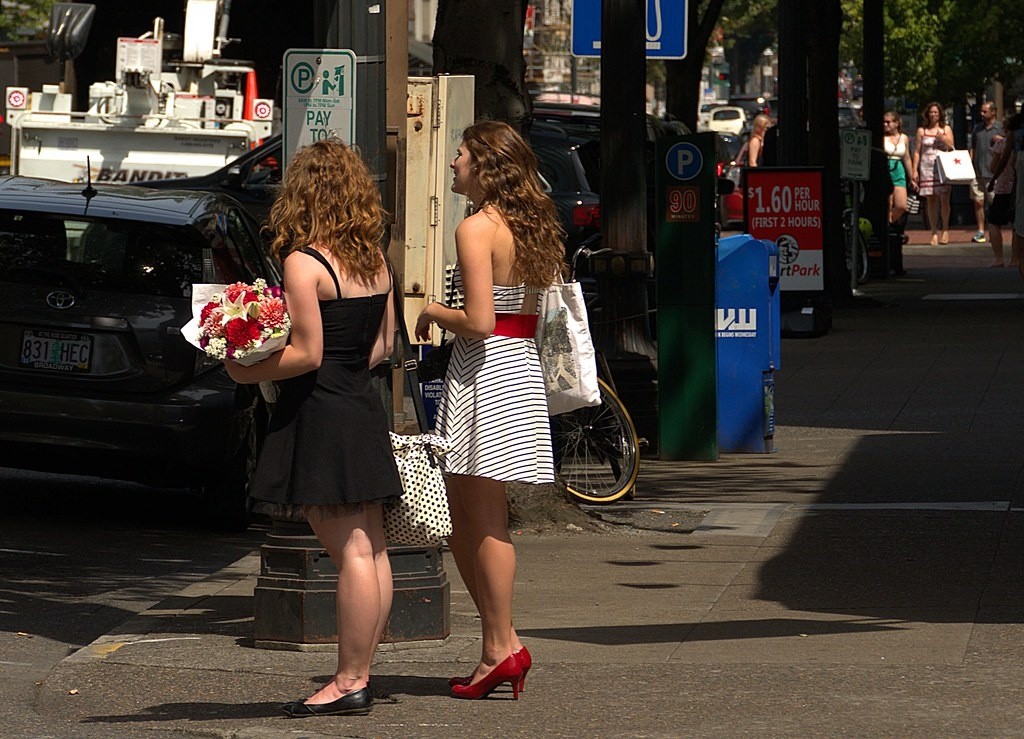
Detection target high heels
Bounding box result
[449,646,532,700]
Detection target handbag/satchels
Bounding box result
[380,430,453,547]
[535,266,603,414]
[936,145,976,185]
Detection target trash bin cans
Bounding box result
[713,232,781,455]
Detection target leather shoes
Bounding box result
[282,680,374,716]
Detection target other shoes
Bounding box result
[929,230,950,245]
[971,230,986,243]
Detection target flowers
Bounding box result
[181,278,293,402]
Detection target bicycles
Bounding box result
[553,233,658,506]
[840,178,868,284]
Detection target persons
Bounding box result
[858,102,955,246]
[747,114,778,167]
[223,136,405,716]
[414,123,569,700]
[969,100,1024,278]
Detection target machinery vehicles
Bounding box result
[0,0,281,253]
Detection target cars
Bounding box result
[75,95,778,272]
[1,172,300,540]
